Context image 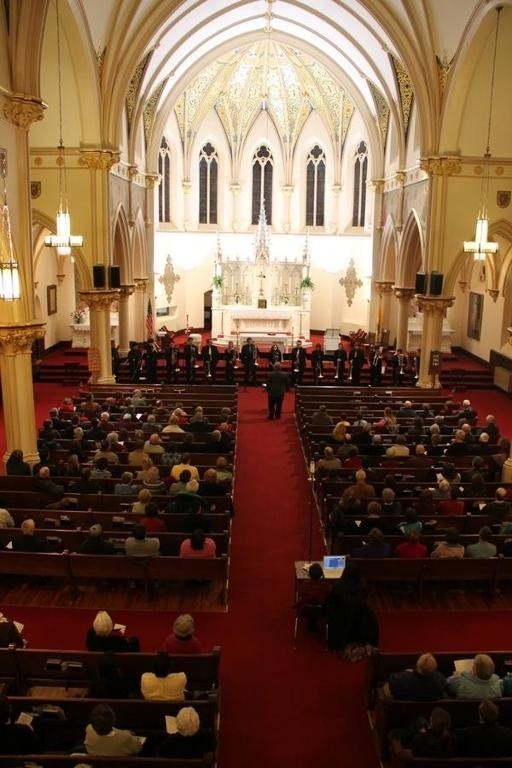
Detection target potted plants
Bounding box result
[209,274,224,307]
[299,276,317,312]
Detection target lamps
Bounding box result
[464,2,504,261]
[45,0,84,255]
[0,147,21,300]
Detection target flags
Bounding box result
[146,299,153,338]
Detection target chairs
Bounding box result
[324,329,341,354]
[292,606,330,648]
[349,328,390,351]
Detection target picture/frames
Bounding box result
[47,284,57,316]
[467,292,484,342]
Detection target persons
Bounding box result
[2,389,233,590]
[381,653,511,759]
[2,611,218,759]
[292,563,379,647]
[294,391,512,599]
[126,338,420,419]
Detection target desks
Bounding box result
[239,333,289,354]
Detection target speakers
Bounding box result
[93,264,105,287]
[110,266,120,288]
[416,272,426,295]
[430,272,443,295]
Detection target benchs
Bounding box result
[291,383,512,594]
[4,382,239,602]
[362,649,511,768]
[0,644,223,765]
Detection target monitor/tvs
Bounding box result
[323,556,346,568]
